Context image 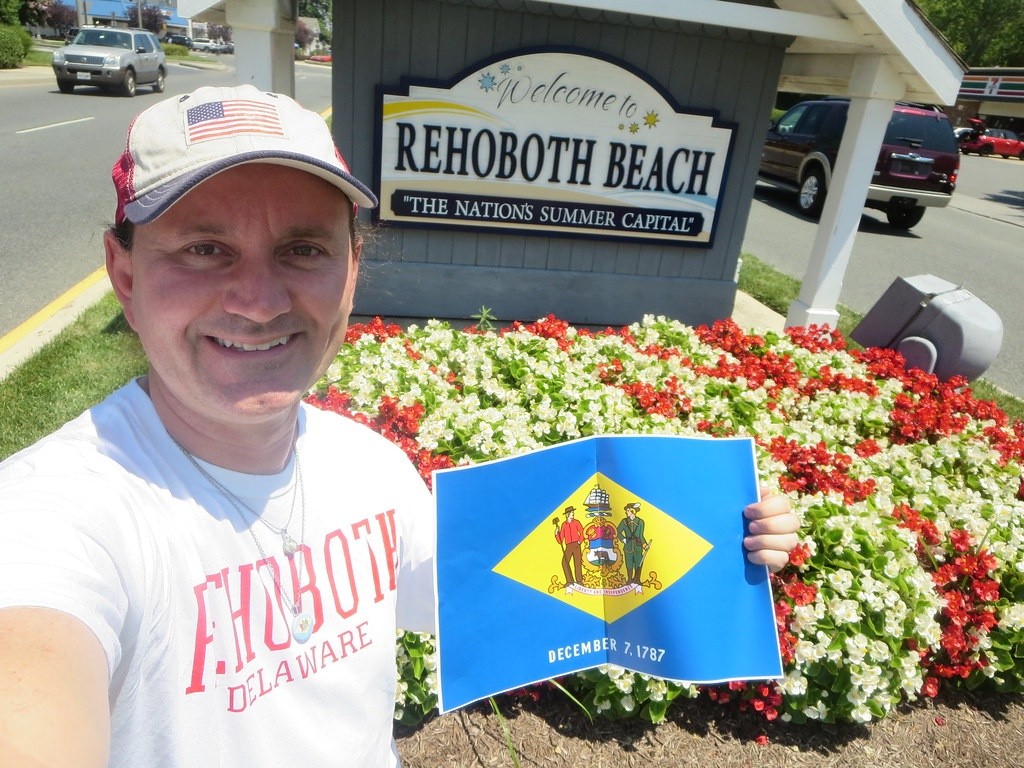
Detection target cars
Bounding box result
[162,33,234,55]
[62,27,81,46]
[954,117,1024,162]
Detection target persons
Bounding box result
[0,84,801,768]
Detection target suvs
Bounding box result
[51,24,169,98]
[755,97,962,228]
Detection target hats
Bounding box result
[113,84,378,231]
